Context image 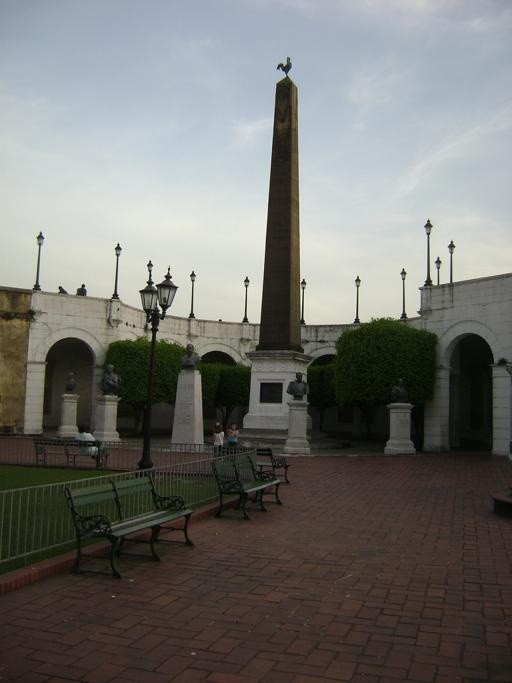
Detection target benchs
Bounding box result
[65,473,194,579]
[211,453,282,521]
[214,445,291,483]
[33,436,109,468]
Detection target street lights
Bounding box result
[434,256,441,285]
[243,276,250,322]
[424,218,433,286]
[189,270,197,317]
[447,240,455,283]
[112,242,122,298]
[354,275,361,323]
[33,231,45,290]
[147,260,153,280]
[400,268,406,318]
[137,263,177,478]
[300,278,306,324]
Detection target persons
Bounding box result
[225,422,239,454]
[213,421,225,456]
[75,423,106,465]
